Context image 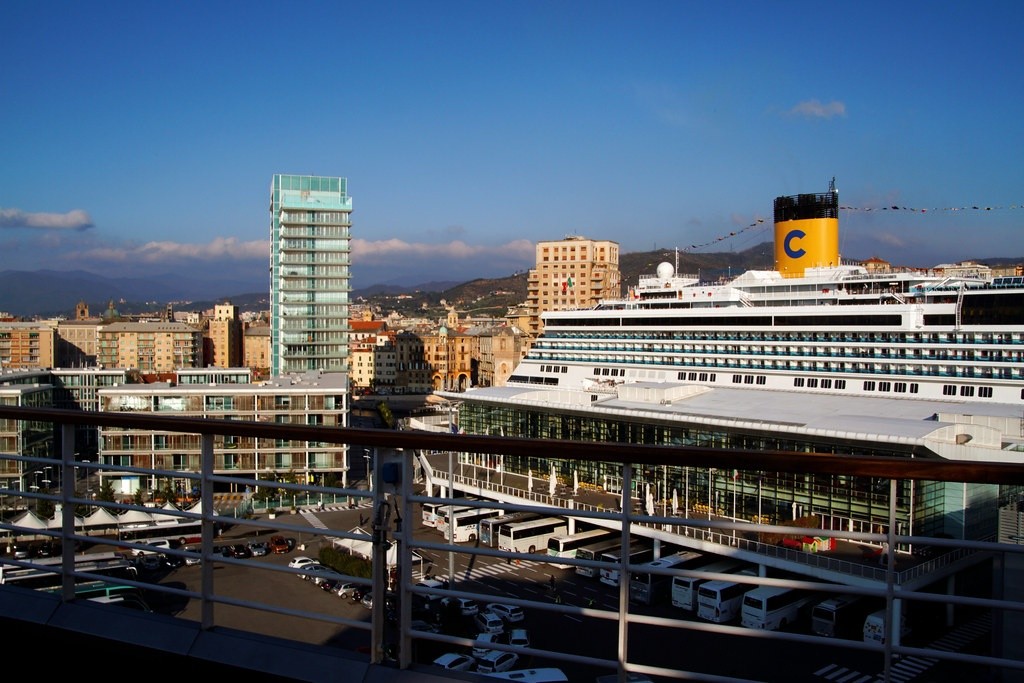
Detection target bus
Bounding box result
[421,494,569,555]
[575,535,641,578]
[118,517,205,547]
[671,561,744,611]
[545,527,616,569]
[598,543,667,588]
[697,569,759,623]
[0,551,157,615]
[480,665,568,683]
[741,585,816,633]
[629,549,704,606]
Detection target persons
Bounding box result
[507,549,511,564]
[550,575,556,589]
[317,500,322,511]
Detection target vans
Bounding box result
[595,671,655,683]
[863,605,913,644]
[810,595,860,639]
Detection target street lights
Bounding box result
[0,451,91,513]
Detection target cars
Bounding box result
[143,535,289,569]
[505,628,532,650]
[472,632,499,658]
[287,556,394,611]
[409,580,478,637]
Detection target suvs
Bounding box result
[475,650,520,677]
[130,538,172,557]
[486,601,526,623]
[432,651,477,672]
[474,609,505,635]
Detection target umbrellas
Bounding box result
[528,469,533,498]
[645,484,654,516]
[620,489,623,513]
[573,470,578,495]
[549,466,557,499]
[673,488,678,517]
